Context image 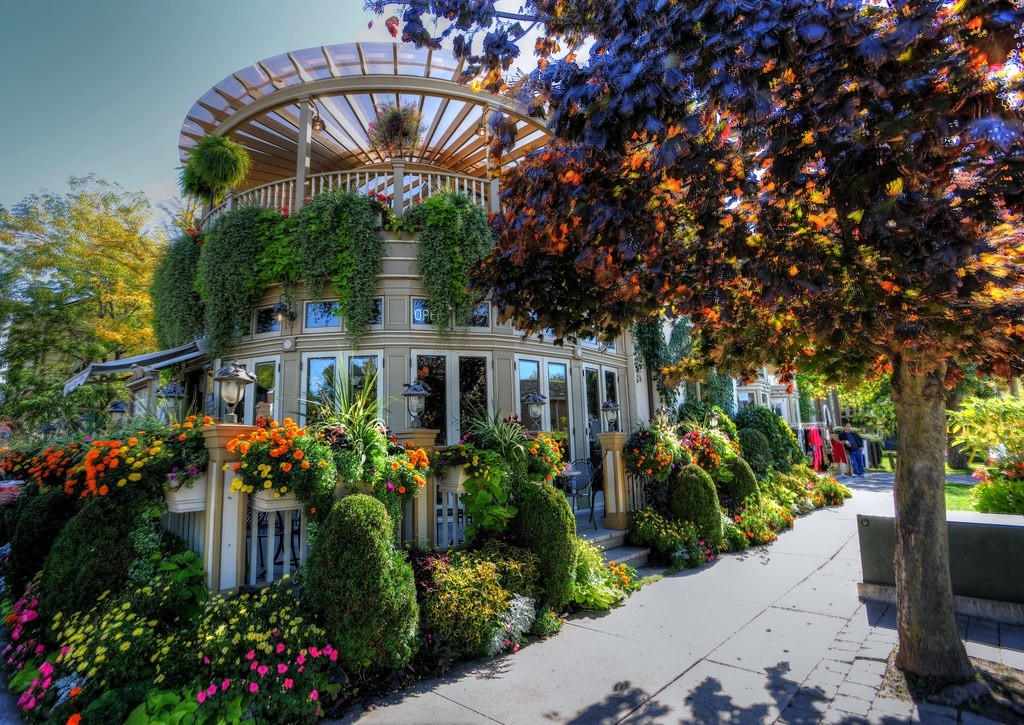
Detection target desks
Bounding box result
[560,471,582,493]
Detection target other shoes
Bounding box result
[852,473,857,477]
[860,474,865,476]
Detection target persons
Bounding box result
[839,423,868,476]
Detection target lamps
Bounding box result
[212,357,257,424]
[271,293,297,323]
[600,398,621,433]
[475,114,486,136]
[401,376,431,429]
[524,389,548,431]
[155,381,188,413]
[707,410,719,427]
[655,403,672,423]
[312,105,326,133]
[107,398,129,424]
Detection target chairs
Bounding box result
[564,459,597,531]
[244,495,298,585]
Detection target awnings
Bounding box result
[63,341,199,395]
